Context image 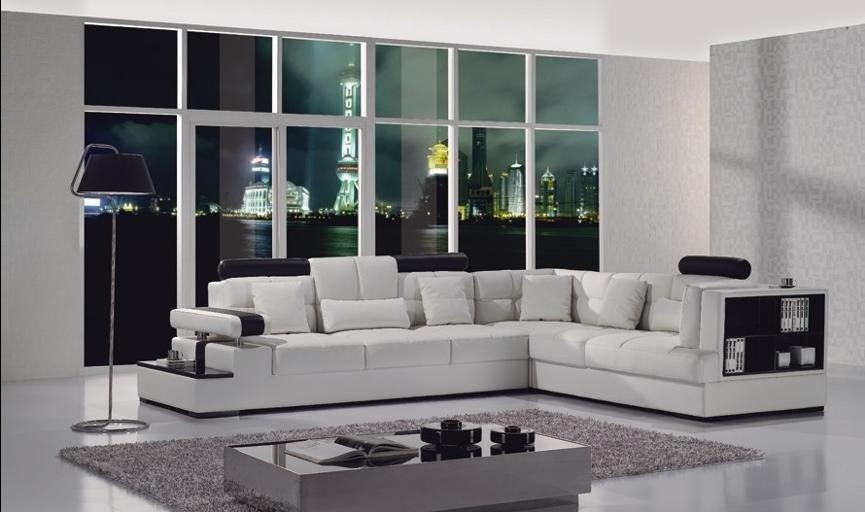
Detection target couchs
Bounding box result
[133,254,830,423]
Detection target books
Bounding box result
[283,434,419,464]
[724,337,746,375]
[780,297,810,334]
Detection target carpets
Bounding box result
[56,406,768,512]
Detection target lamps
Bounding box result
[70,143,154,434]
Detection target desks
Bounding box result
[225,420,593,512]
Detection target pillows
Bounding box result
[416,276,473,326]
[518,274,573,322]
[250,279,310,335]
[648,298,682,333]
[318,296,411,332]
[595,278,646,331]
